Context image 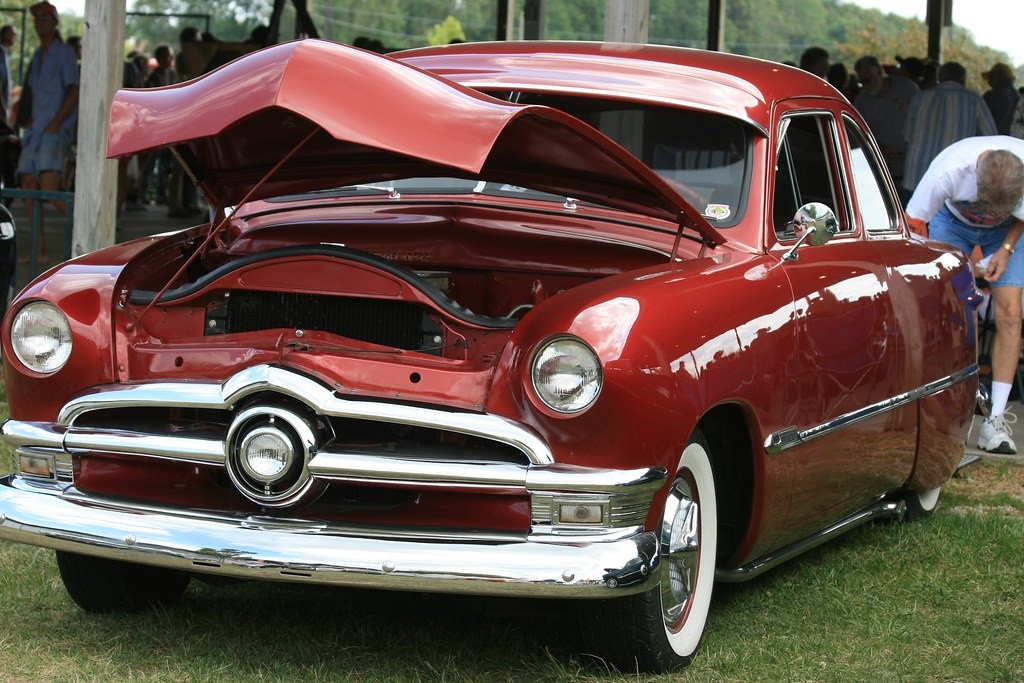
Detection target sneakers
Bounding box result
[977,405,1018,454]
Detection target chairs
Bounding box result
[714,159,785,220]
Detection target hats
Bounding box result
[894,54,925,82]
[29,2,59,23]
[980,62,1016,84]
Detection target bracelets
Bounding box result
[1001,242,1015,255]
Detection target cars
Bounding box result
[0,38,982,678]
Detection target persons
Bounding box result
[16,1,79,264]
[0,22,463,219]
[979,63,1024,139]
[781,46,940,197]
[905,133,1024,455]
[901,61,997,207]
